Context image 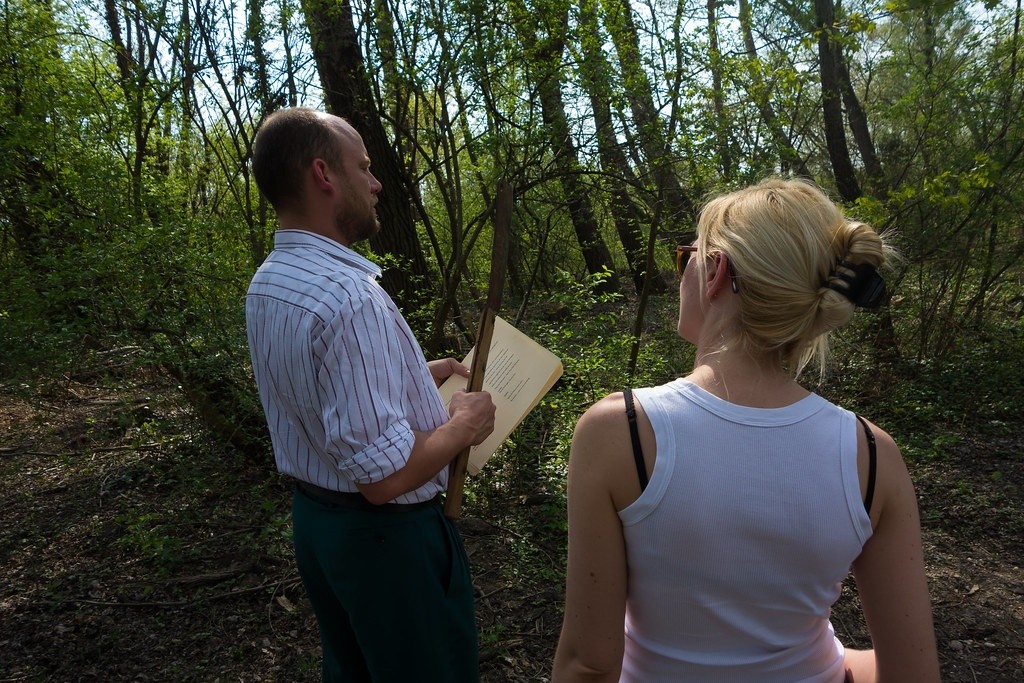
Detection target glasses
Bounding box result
[676,245,738,294]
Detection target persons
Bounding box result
[553,180,942,683]
[245,108,497,683]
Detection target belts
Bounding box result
[299,481,442,512]
